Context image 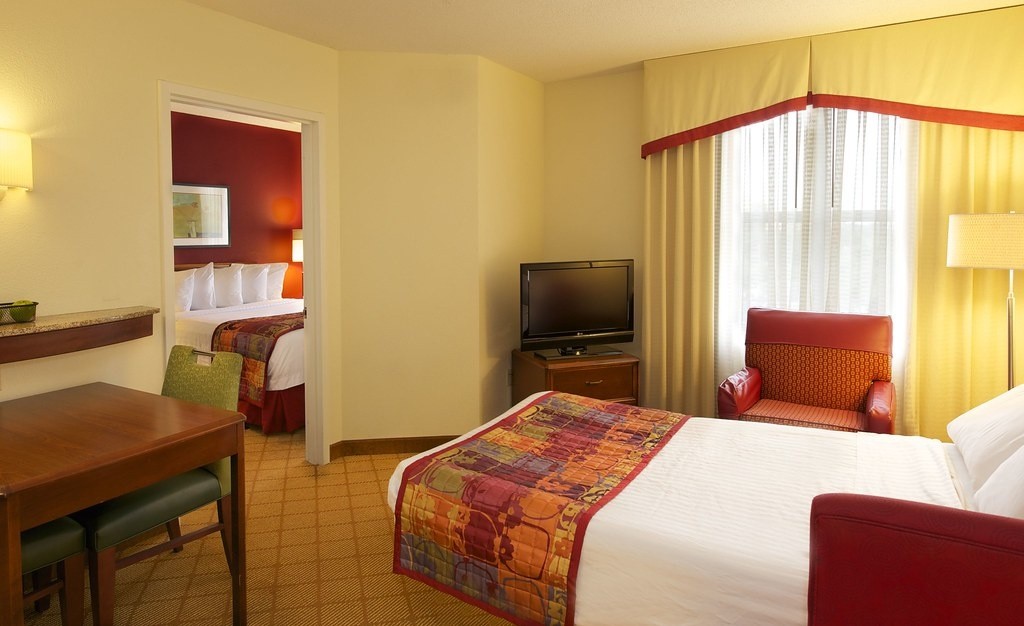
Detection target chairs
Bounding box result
[0,345,245,626]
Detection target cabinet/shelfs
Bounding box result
[510,344,641,407]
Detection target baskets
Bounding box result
[0,302,39,325]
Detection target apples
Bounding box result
[9,299,36,323]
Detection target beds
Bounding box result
[386,390,965,626]
[177,299,305,433]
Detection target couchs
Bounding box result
[719,307,898,434]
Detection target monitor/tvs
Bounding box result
[520,259,634,361]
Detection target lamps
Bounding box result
[947,210,1024,390]
[0,127,34,200]
[293,229,303,262]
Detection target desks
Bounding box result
[0,381,247,626]
[0,305,160,365]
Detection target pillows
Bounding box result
[267,263,289,300]
[974,446,1024,519]
[191,261,217,311]
[214,264,246,308]
[174,268,198,311]
[242,264,271,302]
[947,383,1024,482]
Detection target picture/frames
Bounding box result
[172,181,231,249]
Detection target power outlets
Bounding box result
[506,368,512,386]
